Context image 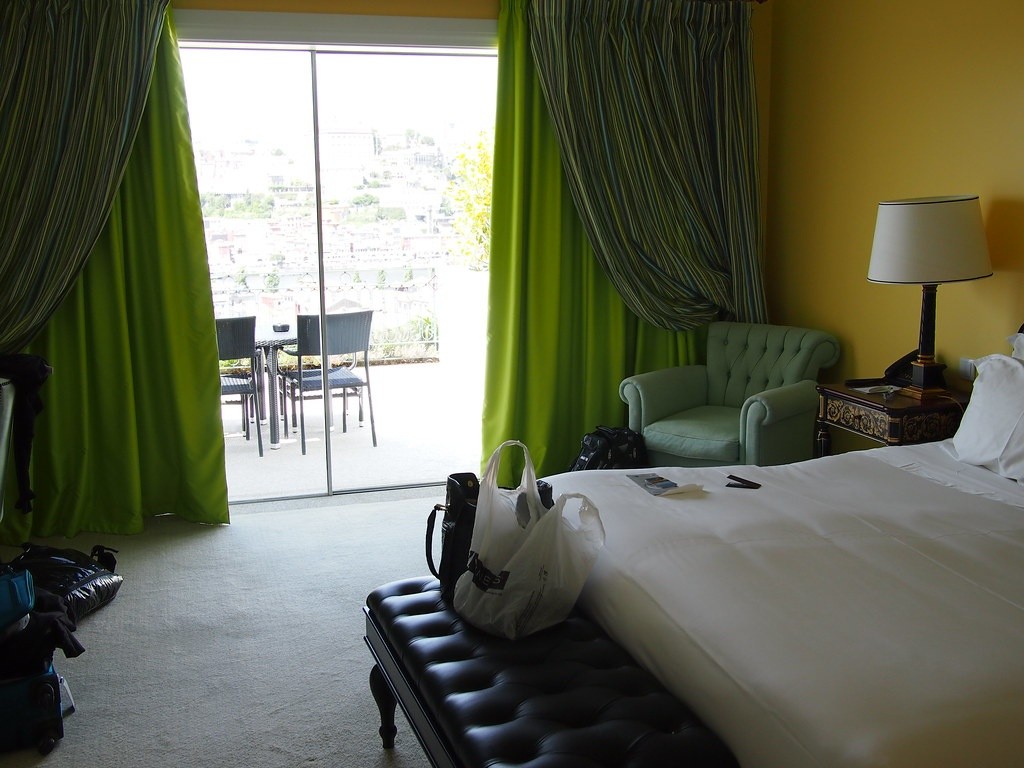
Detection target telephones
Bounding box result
[881,348,946,387]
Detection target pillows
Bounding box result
[941,352,1023,488]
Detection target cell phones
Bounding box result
[646,476,677,489]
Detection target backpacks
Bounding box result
[570,424,649,474]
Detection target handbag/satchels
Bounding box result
[451,441,605,642]
[0,544,123,620]
[424,473,556,603]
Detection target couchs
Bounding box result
[618,322,842,467]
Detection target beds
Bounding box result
[527,322,1024,766]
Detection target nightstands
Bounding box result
[812,378,972,460]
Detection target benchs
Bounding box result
[361,574,744,768]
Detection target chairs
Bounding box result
[282,308,380,452]
[213,316,267,458]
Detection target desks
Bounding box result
[255,320,297,444]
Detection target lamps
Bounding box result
[864,194,994,401]
